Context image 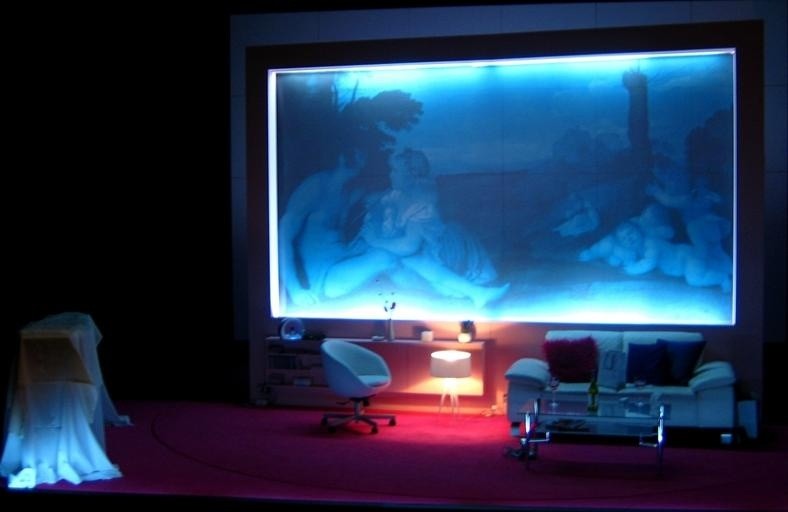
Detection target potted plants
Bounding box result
[457,319,474,343]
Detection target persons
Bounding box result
[605,220,733,295]
[645,169,732,274]
[576,202,678,269]
[277,141,512,308]
[358,149,500,286]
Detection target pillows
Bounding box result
[625,337,707,387]
[540,335,601,383]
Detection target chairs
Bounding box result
[319,339,397,434]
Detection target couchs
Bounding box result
[503,329,739,446]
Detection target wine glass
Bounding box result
[549,376,560,407]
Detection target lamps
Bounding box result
[430,349,473,417]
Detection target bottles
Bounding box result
[588,369,599,410]
